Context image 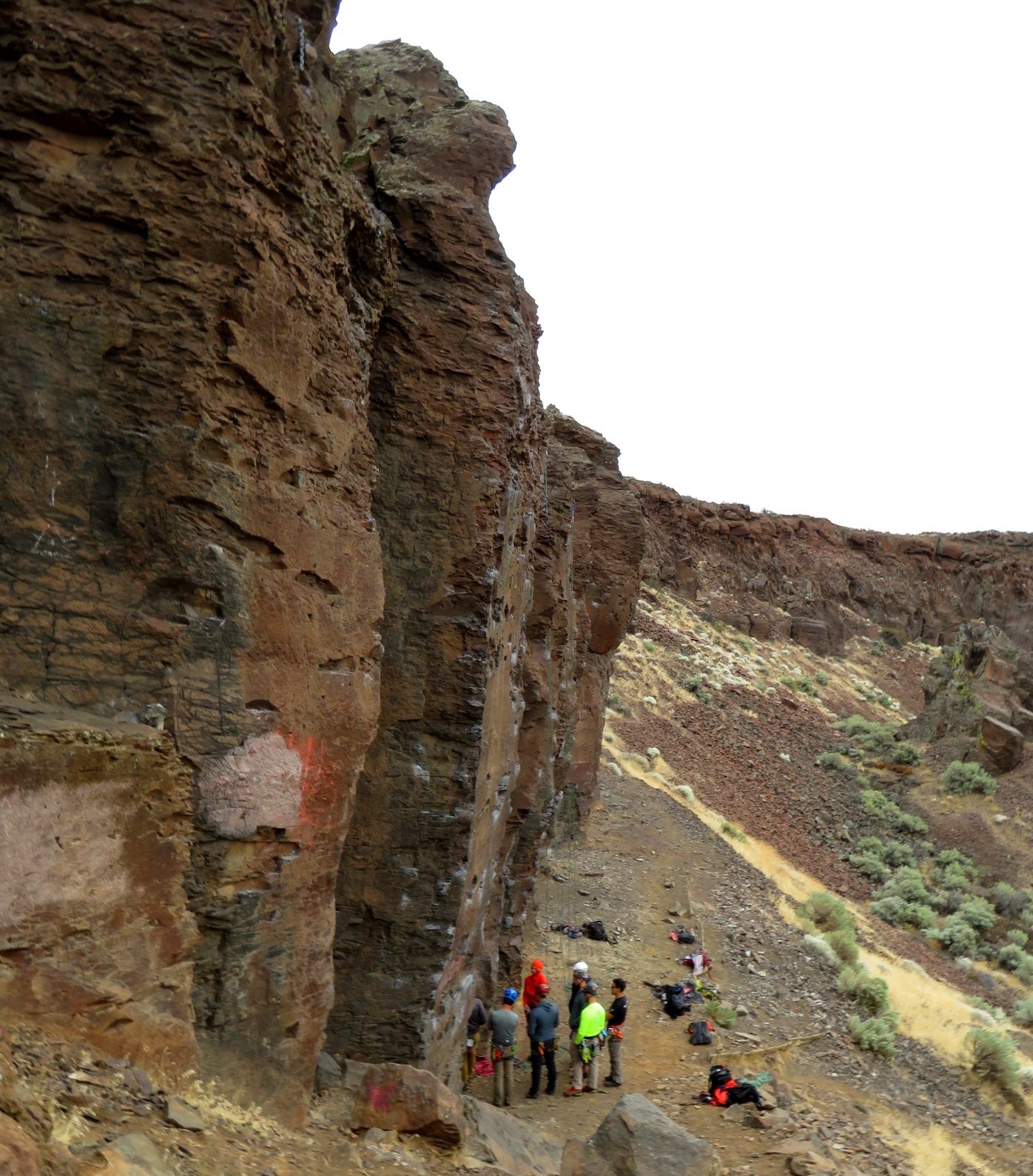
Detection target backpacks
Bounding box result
[588,921,605,941]
[663,982,692,1012]
[708,1069,732,1094]
[725,1082,763,1111]
[689,1021,712,1046]
[678,932,695,944]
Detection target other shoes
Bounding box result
[582,1085,596,1093]
[544,1087,555,1095]
[525,1091,540,1099]
[603,1075,621,1087]
[568,1065,589,1078]
[563,1087,582,1097]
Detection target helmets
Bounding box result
[573,970,592,982]
[580,982,600,995]
[535,984,551,996]
[504,988,518,1001]
[572,962,589,973]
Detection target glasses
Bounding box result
[611,986,616,989]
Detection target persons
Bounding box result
[486,988,519,1106]
[563,962,628,1096]
[525,983,560,1099]
[462,999,487,1090]
[522,958,548,1061]
